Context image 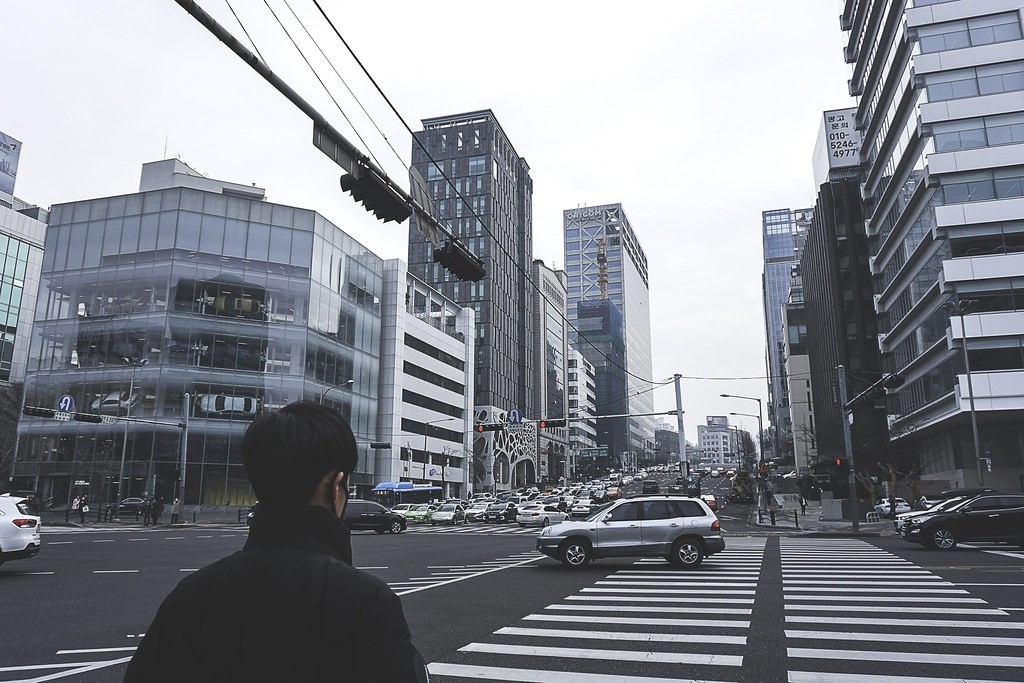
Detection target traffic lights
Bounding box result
[476,423,502,432]
[836,458,848,480]
[24,406,55,417]
[341,173,411,224]
[433,241,485,283]
[75,414,102,422]
[539,421,567,427]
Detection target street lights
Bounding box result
[721,394,767,514]
[730,413,762,485]
[946,299,983,488]
[113,357,147,519]
[709,424,740,472]
[422,417,456,484]
[319,380,354,405]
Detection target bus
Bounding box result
[373,481,443,509]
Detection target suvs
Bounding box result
[894,489,1000,538]
[536,491,728,569]
[901,491,1024,552]
[343,499,408,533]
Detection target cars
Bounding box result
[782,470,805,479]
[483,501,517,523]
[0,493,40,565]
[107,497,146,515]
[689,464,737,510]
[91,391,140,416]
[431,503,464,525]
[102,298,140,313]
[874,497,905,517]
[431,462,681,519]
[214,295,260,315]
[391,502,415,516]
[571,499,598,517]
[199,393,256,415]
[517,503,570,526]
[643,480,660,493]
[405,504,436,523]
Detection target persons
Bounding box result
[122,400,430,683]
[888,495,896,519]
[69,494,88,525]
[800,495,809,517]
[142,496,180,527]
[105,504,121,522]
[920,495,928,511]
